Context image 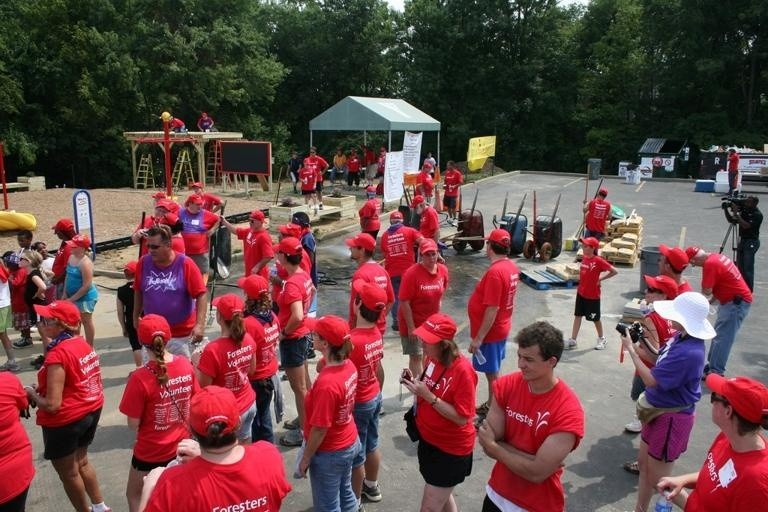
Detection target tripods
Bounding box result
[719,223,739,266]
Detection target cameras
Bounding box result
[399,368,414,384]
[721,189,749,210]
[616,321,644,344]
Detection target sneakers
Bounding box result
[595,338,608,350]
[347,186,353,193]
[447,216,459,226]
[354,184,360,191]
[318,201,325,211]
[0,361,21,372]
[283,416,300,428]
[561,338,577,349]
[361,481,381,502]
[474,403,490,416]
[11,337,33,349]
[625,414,644,432]
[313,203,319,214]
[318,202,326,210]
[280,428,304,448]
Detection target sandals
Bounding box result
[624,459,640,474]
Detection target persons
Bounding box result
[197,292,257,446]
[657,372,768,511]
[656,245,692,295]
[197,112,218,132]
[582,188,612,256]
[161,112,187,132]
[443,160,463,226]
[299,158,317,205]
[0,258,21,371]
[292,212,319,318]
[409,195,440,262]
[423,152,436,180]
[342,279,388,505]
[296,315,363,512]
[132,226,208,345]
[271,237,319,447]
[329,148,347,183]
[272,223,312,275]
[142,385,293,512]
[358,186,381,241]
[61,234,99,350]
[344,233,395,331]
[0,369,36,512]
[398,313,479,512]
[286,151,302,194]
[723,196,763,295]
[303,147,329,210]
[119,313,196,512]
[115,261,145,367]
[724,149,739,198]
[25,299,113,512]
[416,162,434,209]
[621,292,717,512]
[397,238,449,385]
[345,151,362,192]
[686,246,753,381]
[477,321,584,512]
[51,218,76,299]
[360,144,375,168]
[31,242,56,271]
[376,147,387,175]
[157,214,188,258]
[219,210,274,283]
[622,275,678,474]
[191,182,224,213]
[19,250,55,370]
[131,203,169,257]
[7,258,34,349]
[16,230,35,260]
[177,194,220,287]
[236,275,281,444]
[467,229,521,430]
[563,236,618,351]
[152,192,179,213]
[380,212,445,332]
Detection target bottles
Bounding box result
[201,336,210,351]
[655,488,674,511]
[639,298,649,316]
[270,263,277,277]
[165,456,181,467]
[217,257,229,278]
[474,349,486,366]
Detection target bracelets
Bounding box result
[24,386,33,389]
[431,396,439,407]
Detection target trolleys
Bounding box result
[398,183,416,227]
[452,187,485,252]
[523,190,562,262]
[492,191,527,255]
[209,225,231,279]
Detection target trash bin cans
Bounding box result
[637,245,662,294]
[588,158,602,180]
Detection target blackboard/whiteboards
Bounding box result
[218,140,272,178]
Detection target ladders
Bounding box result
[206,141,229,188]
[172,148,194,186]
[137,153,155,189]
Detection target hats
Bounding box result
[151,193,167,199]
[184,194,204,205]
[161,112,174,123]
[159,213,180,226]
[353,279,388,313]
[190,182,204,188]
[409,195,425,208]
[33,302,82,330]
[705,373,768,427]
[420,238,439,254]
[412,313,457,344]
[653,291,717,341]
[138,314,171,346]
[279,222,302,236]
[660,243,689,272]
[189,385,240,439]
[212,293,245,321]
[249,211,269,225]
[292,211,309,225]
[345,234,379,252]
[65,235,91,248]
[237,275,269,300]
[643,273,679,299]
[303,157,316,165]
[599,187,610,196]
[51,219,75,232]
[366,186,376,192]
[423,163,433,169]
[272,236,303,256]
[304,315,352,349]
[685,246,700,262]
[390,211,404,220]
[580,237,600,250]
[155,201,172,211]
[486,229,511,248]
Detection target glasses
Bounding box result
[38,313,58,329]
[70,246,81,251]
[645,286,660,294]
[16,256,33,264]
[145,243,166,249]
[247,218,260,224]
[379,149,386,153]
[706,389,731,411]
[310,151,316,154]
[424,252,438,257]
[692,257,696,267]
[293,153,299,156]
